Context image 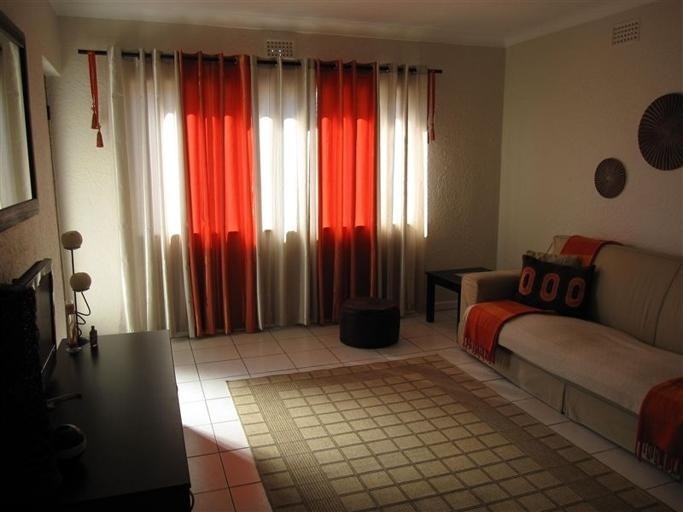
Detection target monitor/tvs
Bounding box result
[12,257,82,413]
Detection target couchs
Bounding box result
[458,236,683,484]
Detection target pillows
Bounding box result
[512,255,596,318]
[526,251,579,266]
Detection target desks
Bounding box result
[1,330,194,511]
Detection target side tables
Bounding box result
[426,267,495,327]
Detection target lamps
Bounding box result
[62,231,92,345]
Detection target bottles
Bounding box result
[89,325,97,347]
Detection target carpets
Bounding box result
[225,353,675,511]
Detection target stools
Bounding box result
[340,298,400,348]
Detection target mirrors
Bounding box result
[0,8,40,234]
